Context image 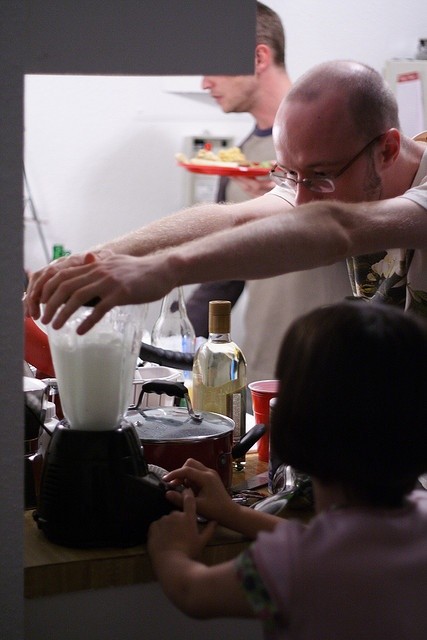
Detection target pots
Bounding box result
[122,381,266,489]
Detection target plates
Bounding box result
[177,150,282,177]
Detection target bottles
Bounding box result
[151,289,195,409]
[192,299,248,471]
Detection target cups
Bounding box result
[248,381,282,463]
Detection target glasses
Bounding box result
[268,132,385,194]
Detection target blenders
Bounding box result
[33,293,171,548]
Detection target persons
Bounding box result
[22,61,427,338]
[181,3,352,414]
[145,300,427,639]
